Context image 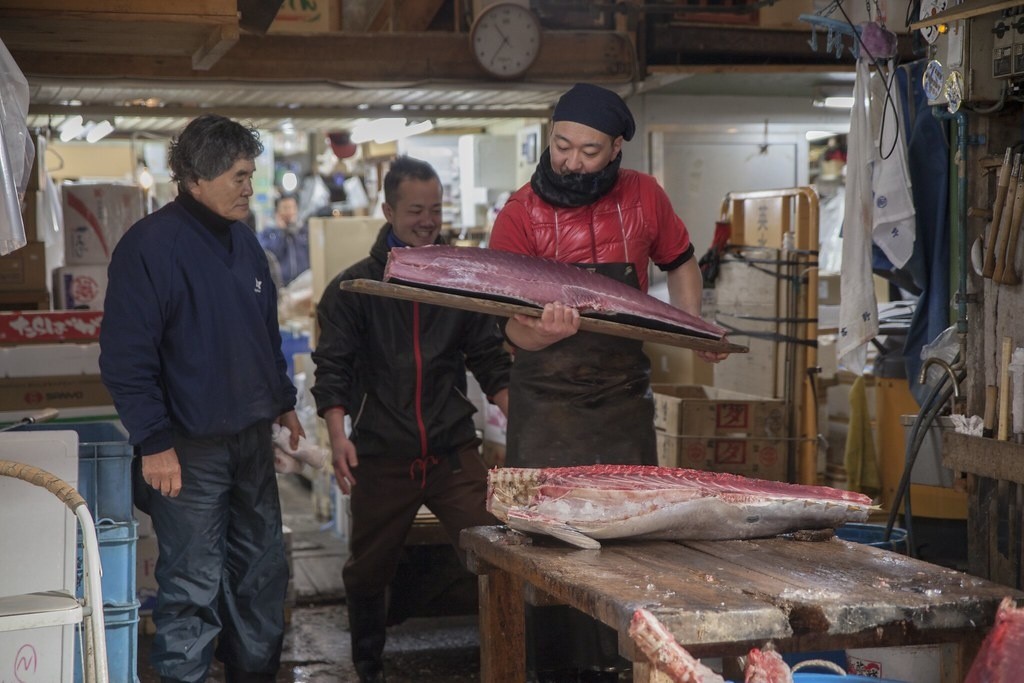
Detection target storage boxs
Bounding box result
[0,423,142,683]
[0,127,149,310]
[308,217,386,303]
[652,383,788,483]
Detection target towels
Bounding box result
[872,60,915,270]
[836,58,879,376]
[272,423,326,474]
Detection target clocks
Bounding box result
[469,2,542,79]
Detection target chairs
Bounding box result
[0,461,109,683]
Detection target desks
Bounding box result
[457,524,1024,683]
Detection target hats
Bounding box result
[553,82,637,142]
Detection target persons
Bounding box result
[101,114,306,683]
[306,153,511,683]
[484,83,731,678]
[249,192,323,392]
